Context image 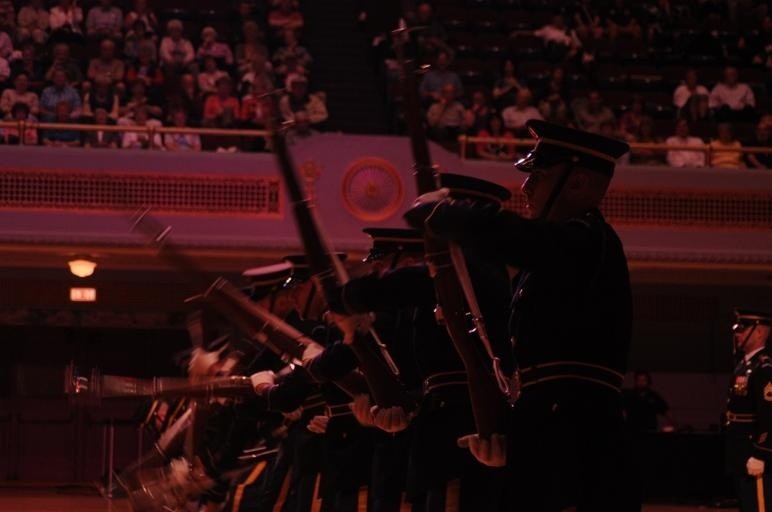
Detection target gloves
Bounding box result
[281,407,304,421]
[457,435,508,467]
[347,395,409,433]
[726,412,753,422]
[189,349,219,377]
[303,343,325,365]
[747,457,764,476]
[306,415,329,434]
[251,371,277,394]
[509,363,625,399]
[170,458,190,484]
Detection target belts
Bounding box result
[323,403,352,417]
[303,395,325,409]
[420,373,465,394]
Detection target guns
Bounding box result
[257,82,417,419]
[68,367,262,404]
[123,205,372,401]
[153,398,204,459]
[401,38,514,441]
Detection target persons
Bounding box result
[720,306,772,512]
[2,2,329,154]
[358,2,772,172]
[620,367,678,504]
[140,119,641,512]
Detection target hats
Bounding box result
[433,172,513,210]
[731,309,772,333]
[134,396,161,430]
[362,227,426,263]
[237,254,347,302]
[514,118,630,173]
